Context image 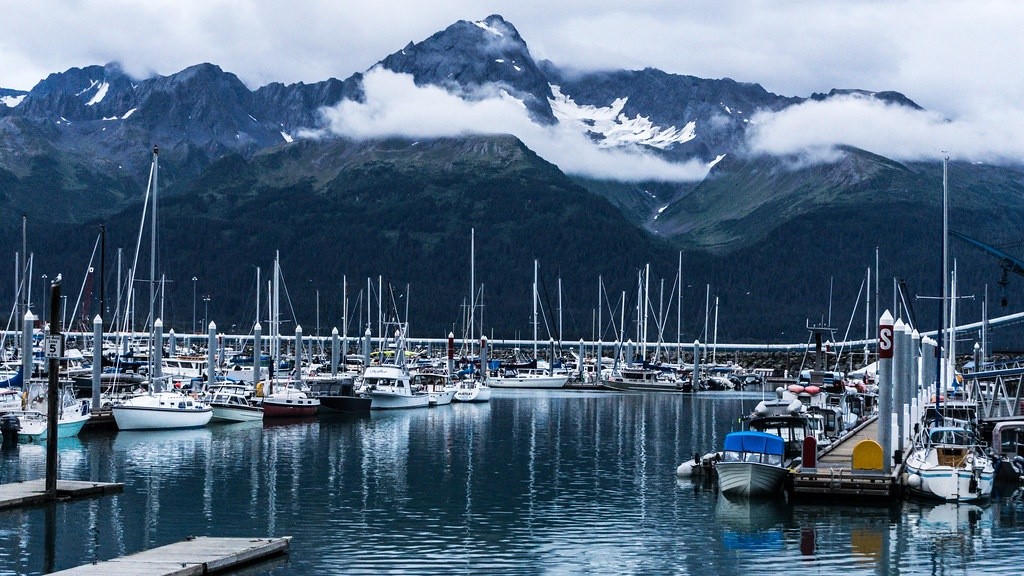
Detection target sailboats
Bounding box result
[0,145,775,444]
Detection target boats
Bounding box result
[670,245,956,499]
[904,150,1024,501]
[715,483,790,555]
